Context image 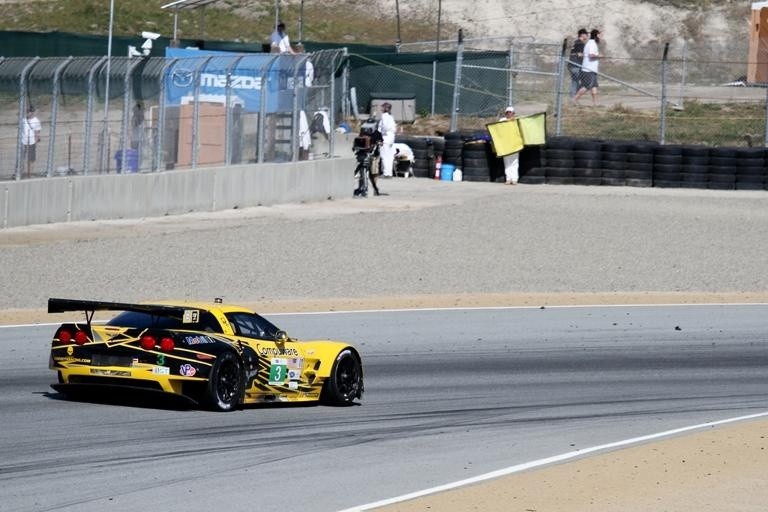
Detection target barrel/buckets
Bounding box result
[440,164,456,180]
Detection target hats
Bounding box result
[578,28,591,34]
[380,103,391,109]
[504,106,515,113]
[590,30,602,36]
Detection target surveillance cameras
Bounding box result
[140,31,161,41]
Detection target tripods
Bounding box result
[354,162,380,197]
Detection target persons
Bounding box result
[360,118,384,195]
[567,28,589,100]
[269,22,295,55]
[377,102,396,179]
[568,29,603,108]
[497,106,520,186]
[129,103,145,150]
[8,104,39,178]
[390,140,415,178]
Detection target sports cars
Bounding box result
[46,296,364,411]
